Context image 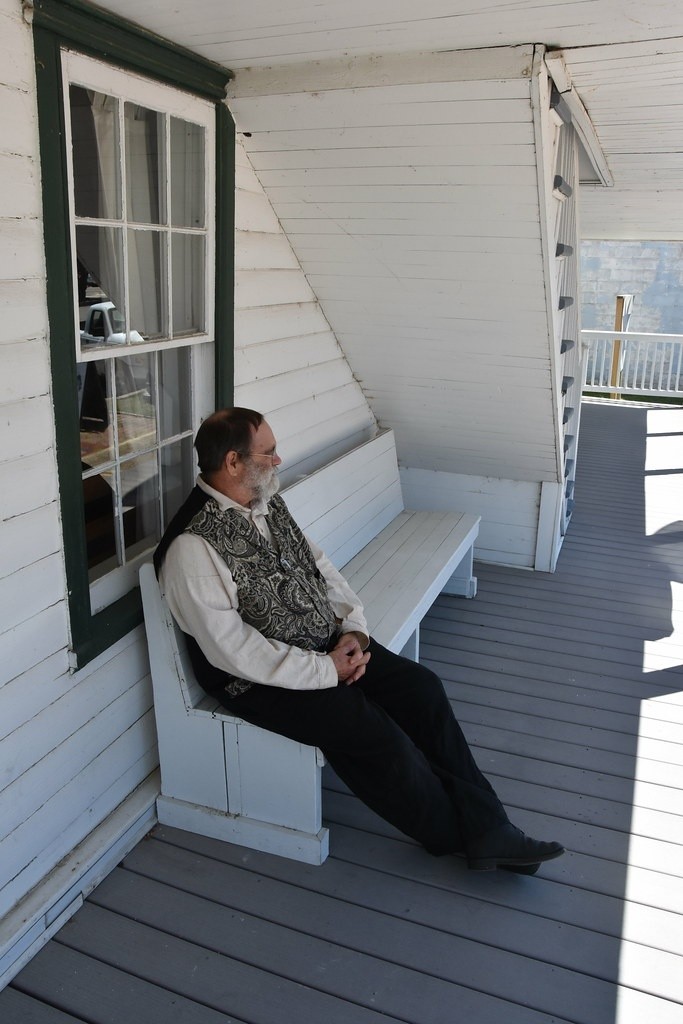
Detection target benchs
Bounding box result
[139,428,480,865]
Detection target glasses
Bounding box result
[251,447,276,465]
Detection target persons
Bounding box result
[154,407,566,876]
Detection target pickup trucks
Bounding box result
[80,302,149,389]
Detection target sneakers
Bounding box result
[467,822,566,876]
